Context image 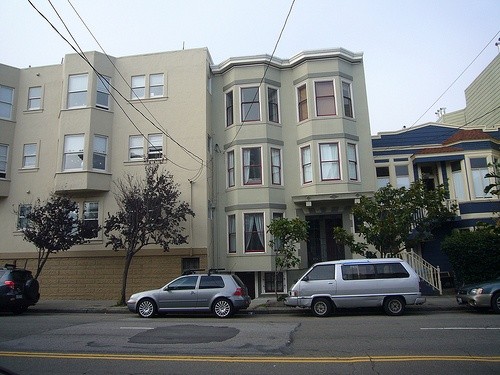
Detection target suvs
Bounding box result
[0,264,40,314]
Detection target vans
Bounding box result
[284,257,427,317]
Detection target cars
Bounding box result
[457,276,500,313]
[127,270,251,320]
